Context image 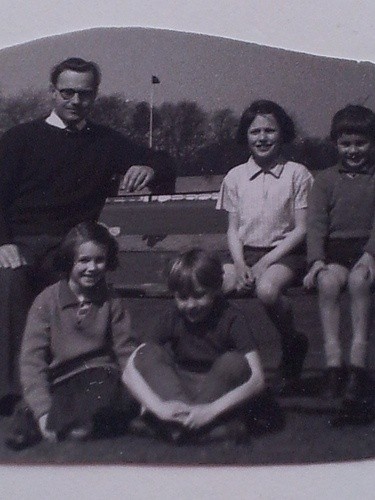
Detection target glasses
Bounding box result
[56,85,94,101]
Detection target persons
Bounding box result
[215,99,317,396]
[122,246,267,446]
[0,57,178,450]
[302,104,375,425]
[7,219,144,451]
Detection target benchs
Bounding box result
[110,232,375,413]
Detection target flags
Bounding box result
[152,76,160,84]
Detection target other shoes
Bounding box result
[127,415,165,440]
[93,408,126,438]
[317,366,342,403]
[274,329,310,386]
[200,418,249,445]
[3,425,42,451]
[342,366,368,406]
